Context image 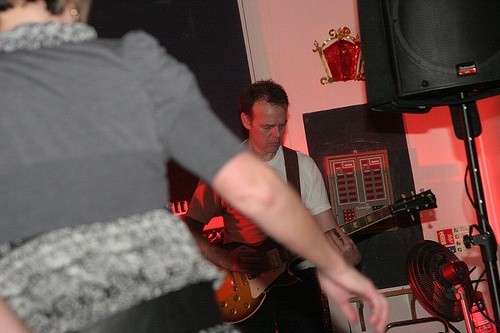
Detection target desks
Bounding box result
[348,287,463,333]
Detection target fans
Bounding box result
[406,240,475,333]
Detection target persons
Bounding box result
[0,0,390,333]
[183,81,361,333]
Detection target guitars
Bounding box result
[211,188,438,324]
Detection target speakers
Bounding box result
[356,0,500,114]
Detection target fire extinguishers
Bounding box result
[468,290,495,333]
